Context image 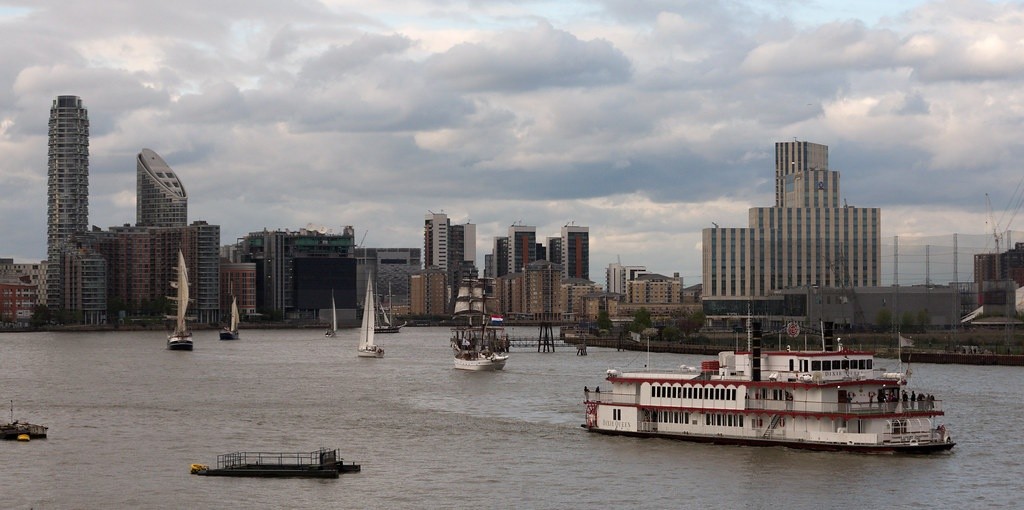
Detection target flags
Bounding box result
[462,338,470,345]
[491,314,504,324]
[900,336,915,347]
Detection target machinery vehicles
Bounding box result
[985,193,1004,281]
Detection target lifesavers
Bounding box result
[191,463,206,471]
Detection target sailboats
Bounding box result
[324,297,337,337]
[450,268,508,370]
[220,296,240,341]
[165,250,194,349]
[358,273,385,357]
[373,281,407,333]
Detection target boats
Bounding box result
[0,401,47,441]
[191,447,360,477]
[583,300,956,452]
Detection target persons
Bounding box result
[847,394,852,411]
[785,392,793,410]
[869,396,874,408]
[595,386,600,393]
[584,386,590,401]
[877,390,935,411]
[745,393,749,409]
[970,347,977,354]
[935,425,946,442]
